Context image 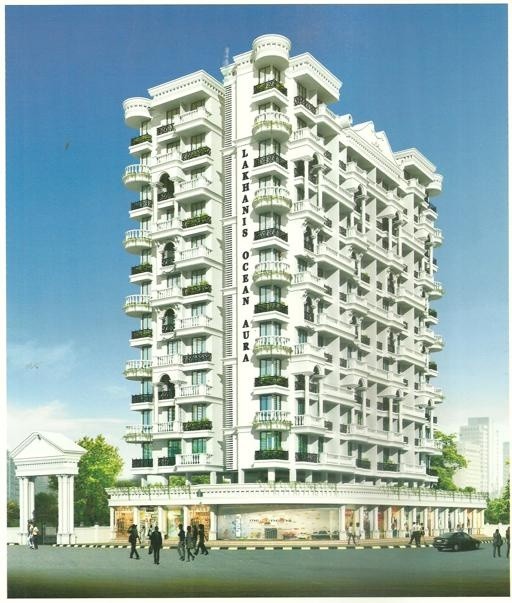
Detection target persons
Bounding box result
[346,516,470,548]
[32,524,41,549]
[162,382,170,397]
[27,522,35,548]
[504,526,509,558]
[492,527,503,557]
[127,521,210,565]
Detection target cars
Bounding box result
[245,528,339,540]
[431,531,483,552]
